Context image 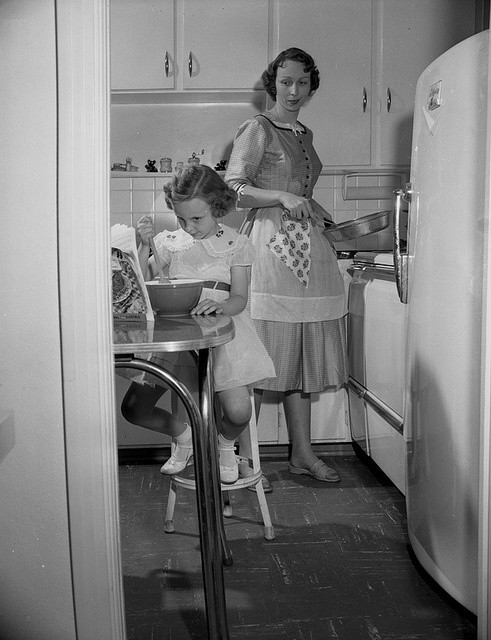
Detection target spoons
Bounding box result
[147,236,171,283]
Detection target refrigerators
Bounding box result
[392,27,486,617]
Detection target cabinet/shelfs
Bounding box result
[116,377,353,450]
[273,1,477,176]
[110,2,272,104]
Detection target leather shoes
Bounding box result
[160,444,194,475]
[219,447,239,483]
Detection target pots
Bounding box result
[141,277,205,318]
[322,208,389,241]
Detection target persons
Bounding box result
[224,49,351,489]
[136,162,256,482]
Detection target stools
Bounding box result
[165,383,278,541]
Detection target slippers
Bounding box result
[239,473,273,492]
[288,459,341,482]
